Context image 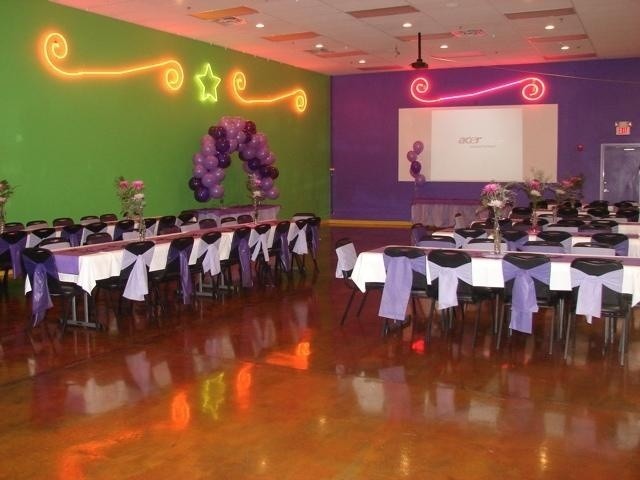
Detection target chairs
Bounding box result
[0,231,29,286]
[308,217,321,272]
[563,258,630,366]
[590,200,609,212]
[53,218,74,226]
[496,253,560,355]
[467,238,507,251]
[616,208,639,222]
[268,221,290,273]
[237,215,254,224]
[591,233,629,256]
[3,222,26,232]
[470,221,485,229]
[114,220,135,241]
[80,216,100,224]
[587,208,609,217]
[157,216,176,236]
[143,219,157,236]
[19,247,85,342]
[572,242,615,256]
[557,219,585,228]
[541,199,556,203]
[157,224,182,236]
[248,224,271,287]
[60,224,85,247]
[559,198,582,208]
[590,220,618,232]
[83,222,107,246]
[198,218,217,230]
[557,207,579,217]
[382,246,430,336]
[151,236,194,322]
[614,202,633,212]
[500,230,530,242]
[30,228,56,248]
[26,220,50,228]
[455,213,466,228]
[529,201,548,210]
[427,249,495,349]
[536,231,572,248]
[100,214,118,221]
[192,231,222,301]
[93,241,156,333]
[522,218,549,226]
[220,217,237,226]
[462,218,465,219]
[178,210,199,226]
[485,217,512,229]
[409,223,427,246]
[33,237,72,248]
[87,232,113,245]
[292,213,315,220]
[220,227,252,294]
[568,224,572,224]
[453,229,487,243]
[512,207,533,214]
[516,241,566,252]
[417,235,456,248]
[334,237,385,326]
[288,219,308,272]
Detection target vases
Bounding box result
[552,207,559,223]
[530,210,539,229]
[493,221,502,254]
[570,201,576,208]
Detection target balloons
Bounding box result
[188,178,202,191]
[219,114,232,132]
[202,173,218,188]
[410,160,421,173]
[209,184,225,199]
[228,139,238,154]
[217,154,232,168]
[413,140,424,154]
[407,150,416,162]
[215,138,229,152]
[192,152,205,165]
[210,168,225,183]
[191,164,208,178]
[409,168,420,180]
[202,144,217,157]
[209,125,226,139]
[199,134,214,145]
[266,186,279,201]
[233,116,280,191]
[203,155,218,171]
[226,124,239,140]
[194,186,210,203]
[415,174,425,186]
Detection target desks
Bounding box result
[198,204,281,222]
[410,197,512,228]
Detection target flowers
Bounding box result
[475,184,518,220]
[521,177,544,209]
[549,179,570,207]
[566,176,582,201]
[116,176,148,240]
[0,179,14,232]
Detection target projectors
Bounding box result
[410,61,430,72]
